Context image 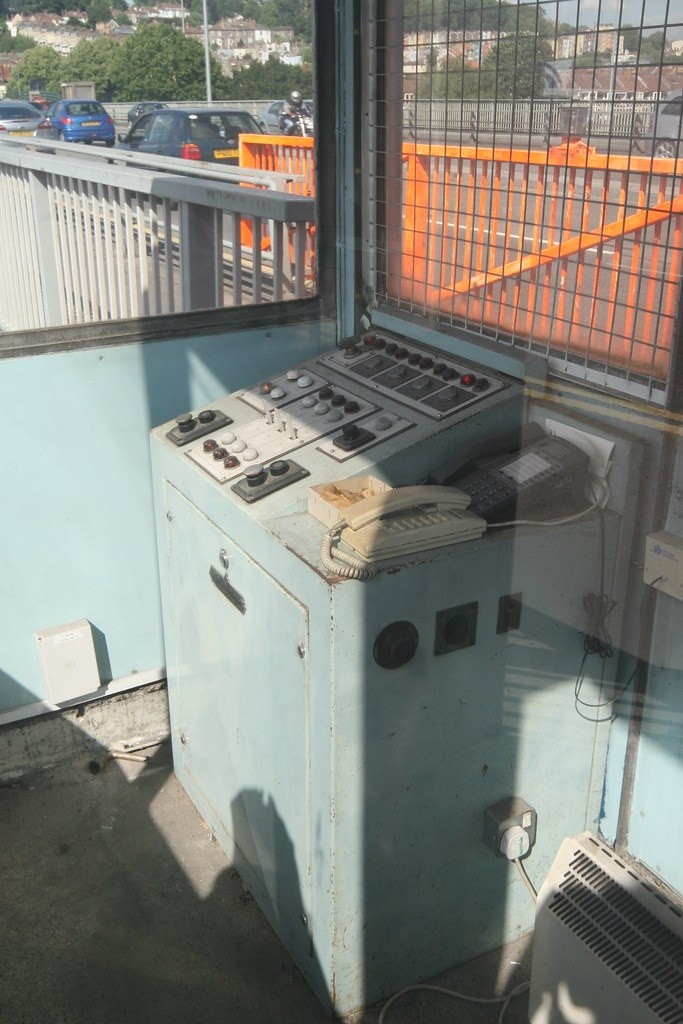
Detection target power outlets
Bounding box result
[484,796,537,860]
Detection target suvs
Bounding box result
[644,89,683,160]
[107,109,268,210]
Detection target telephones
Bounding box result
[336,484,488,564]
[429,420,591,523]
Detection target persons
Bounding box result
[277,91,312,136]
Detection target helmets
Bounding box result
[290,91,301,105]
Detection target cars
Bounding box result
[128,103,169,128]
[256,100,314,136]
[43,99,116,147]
[0,101,58,148]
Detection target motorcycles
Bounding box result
[282,113,310,138]
